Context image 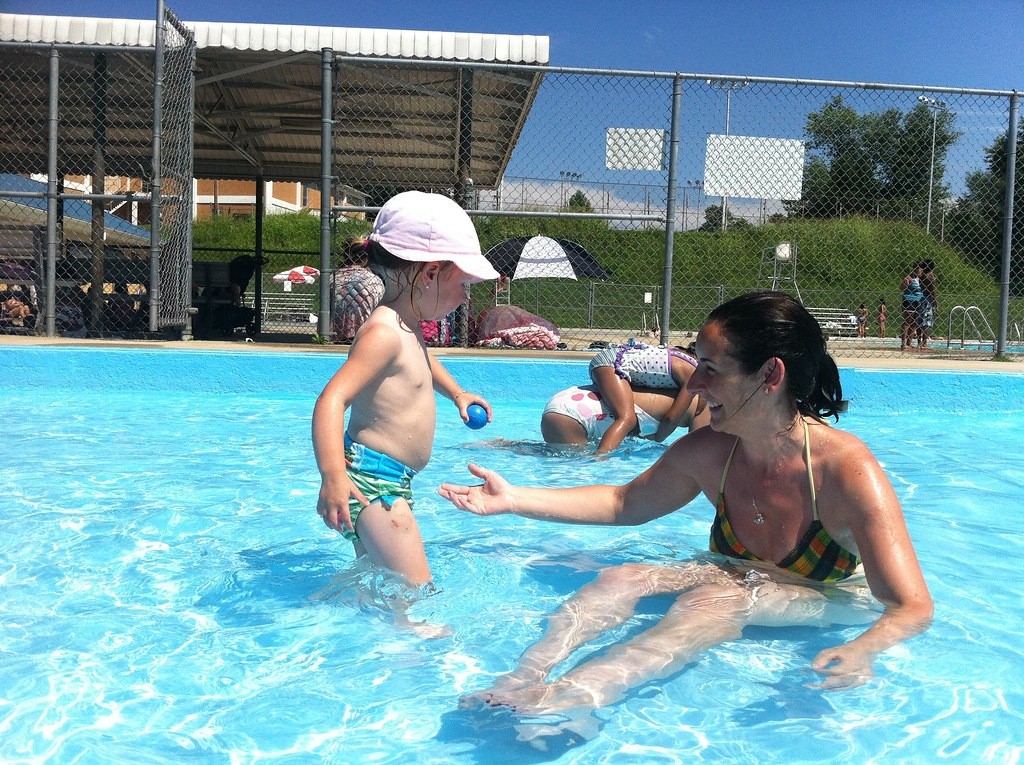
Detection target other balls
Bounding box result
[464,404,487,431]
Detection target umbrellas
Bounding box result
[274,264,320,294]
[484,234,614,317]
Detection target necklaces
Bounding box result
[750,496,766,525]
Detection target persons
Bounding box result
[329,236,388,343]
[858,304,866,338]
[467,384,712,462]
[900,262,923,350]
[1,295,31,319]
[434,290,934,723]
[877,298,888,338]
[919,259,938,350]
[587,342,703,450]
[313,190,493,586]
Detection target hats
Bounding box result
[367,190,500,283]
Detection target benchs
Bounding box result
[805,308,869,337]
[0,222,80,337]
[68,258,230,340]
[241,292,316,322]
